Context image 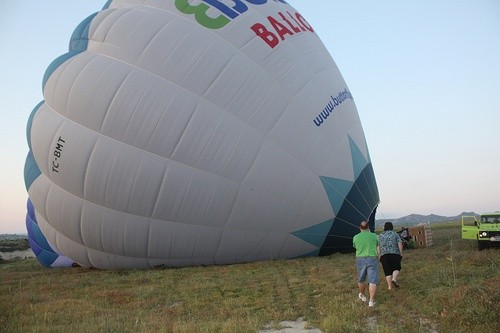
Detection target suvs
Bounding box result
[457,212,500,253]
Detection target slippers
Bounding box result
[392,280,399,288]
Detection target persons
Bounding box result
[400,227,408,250]
[379,222,402,292]
[353,221,380,306]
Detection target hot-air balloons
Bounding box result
[23,0,435,269]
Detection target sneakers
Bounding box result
[359,292,367,301]
[369,301,376,307]
